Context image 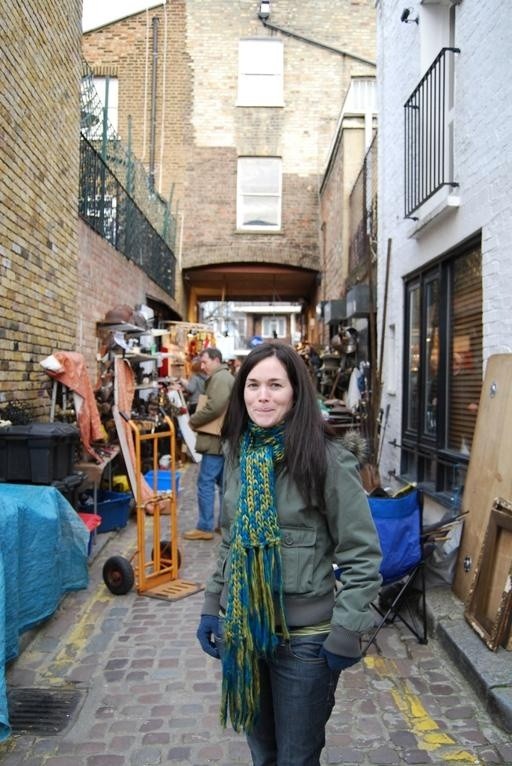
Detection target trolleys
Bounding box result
[101,404,204,601]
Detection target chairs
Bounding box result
[335,489,429,655]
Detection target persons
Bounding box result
[187,347,235,540]
[233,353,244,373]
[182,360,205,414]
[196,342,385,766]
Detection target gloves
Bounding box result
[197,615,220,658]
[318,647,360,670]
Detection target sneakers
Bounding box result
[183,529,214,540]
